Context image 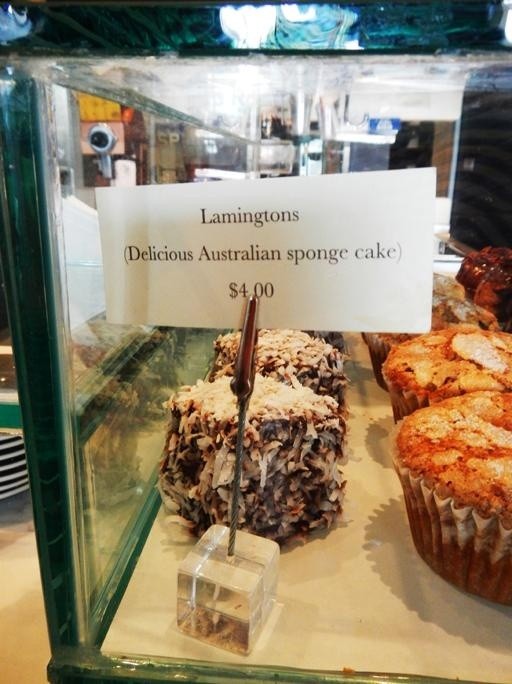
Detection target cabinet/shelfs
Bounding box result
[1,0,511,683]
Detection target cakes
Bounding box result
[381,327,512,425]
[385,387,512,608]
[205,328,353,412]
[366,296,503,392]
[158,372,348,550]
[432,271,466,301]
